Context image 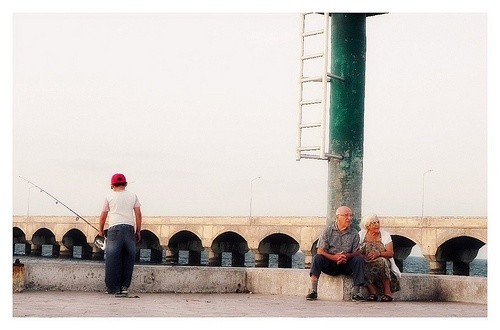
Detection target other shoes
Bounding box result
[381,295,392,302]
[352,294,365,302]
[306,290,317,299]
[367,293,377,300]
[104,289,116,294]
[117,286,128,293]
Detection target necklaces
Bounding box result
[370,234,378,240]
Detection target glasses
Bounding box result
[339,214,353,218]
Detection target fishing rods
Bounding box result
[18,176,106,236]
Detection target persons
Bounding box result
[99,173,141,294]
[358,213,401,301]
[306,206,366,302]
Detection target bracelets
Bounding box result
[380,252,381,256]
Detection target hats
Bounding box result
[111,173,126,189]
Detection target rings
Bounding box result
[373,255,374,257]
